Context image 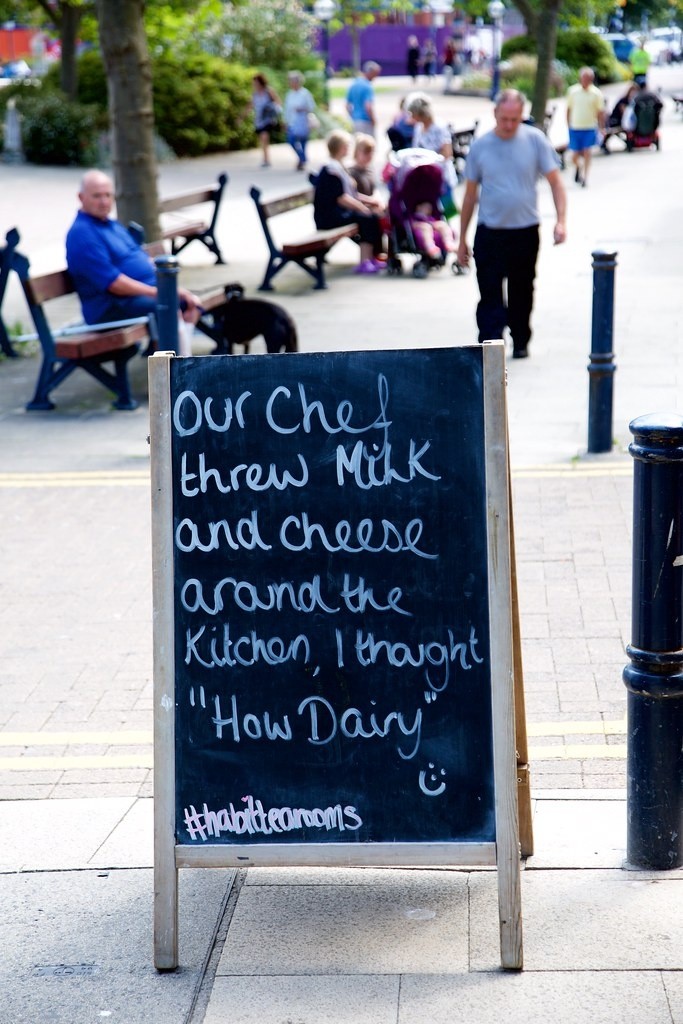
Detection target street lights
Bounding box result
[314,0,337,112]
[488,0,505,100]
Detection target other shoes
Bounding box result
[374,260,387,268]
[512,341,528,358]
[361,259,376,272]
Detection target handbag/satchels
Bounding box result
[307,113,322,128]
[270,122,285,143]
[621,98,637,130]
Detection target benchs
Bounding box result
[539,95,613,169]
[249,187,362,291]
[448,122,480,174]
[5,227,242,416]
[154,175,228,265]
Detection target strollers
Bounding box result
[626,93,662,150]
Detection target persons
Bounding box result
[609,41,663,148]
[281,69,317,170]
[237,74,282,168]
[346,61,381,138]
[457,88,566,359]
[313,90,458,275]
[407,35,457,84]
[65,167,202,358]
[566,67,606,189]
